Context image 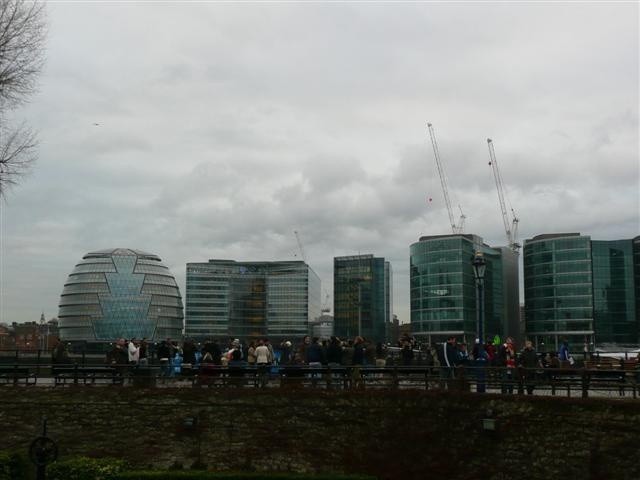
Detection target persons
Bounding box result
[53,343,70,382]
[101,334,573,398]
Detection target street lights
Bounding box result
[470,249,490,393]
